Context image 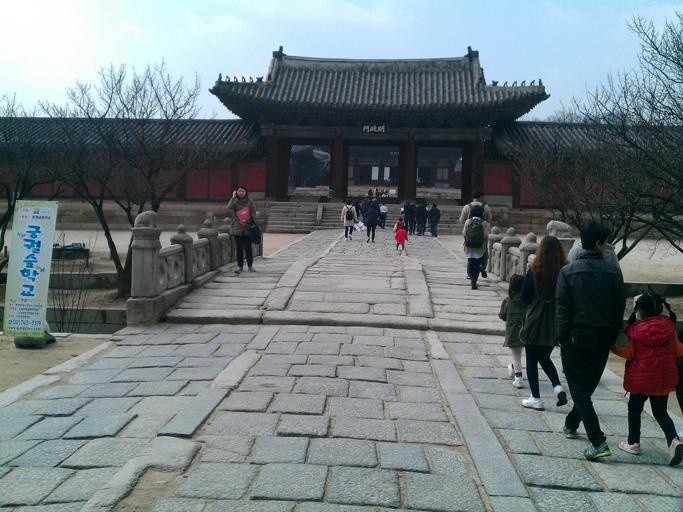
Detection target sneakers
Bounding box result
[522,395,544,411]
[563,424,576,438]
[512,376,524,388]
[583,441,612,459]
[508,363,516,377]
[619,440,641,455]
[667,438,683,466]
[554,385,568,406]
[367,237,375,243]
[346,234,352,241]
[247,266,255,272]
[234,266,243,273]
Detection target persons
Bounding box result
[227,186,256,274]
[460,191,493,289]
[341,185,441,251]
[520,236,568,411]
[555,223,626,460]
[611,291,683,465]
[500,274,526,388]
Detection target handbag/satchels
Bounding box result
[251,224,261,244]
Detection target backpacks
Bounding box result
[346,205,354,220]
[465,218,486,248]
[468,203,489,218]
